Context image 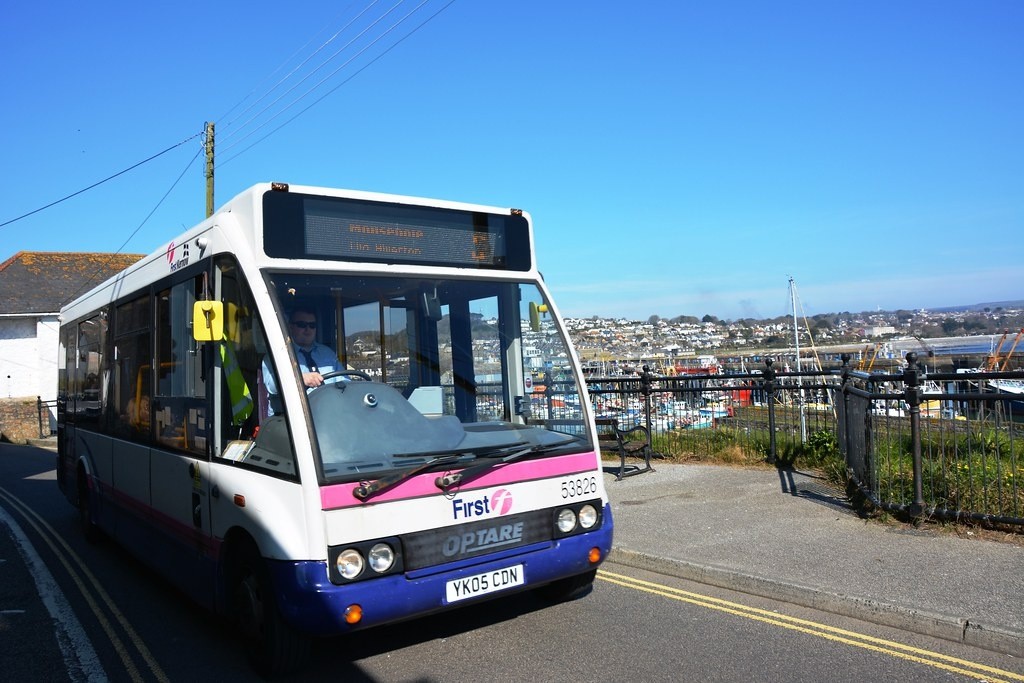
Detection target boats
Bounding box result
[440,274,1023,441]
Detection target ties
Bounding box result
[299,348,325,388]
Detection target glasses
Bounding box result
[291,321,316,328]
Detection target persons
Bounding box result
[263,305,353,421]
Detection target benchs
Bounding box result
[527,417,655,481]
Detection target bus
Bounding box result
[53,179,622,642]
